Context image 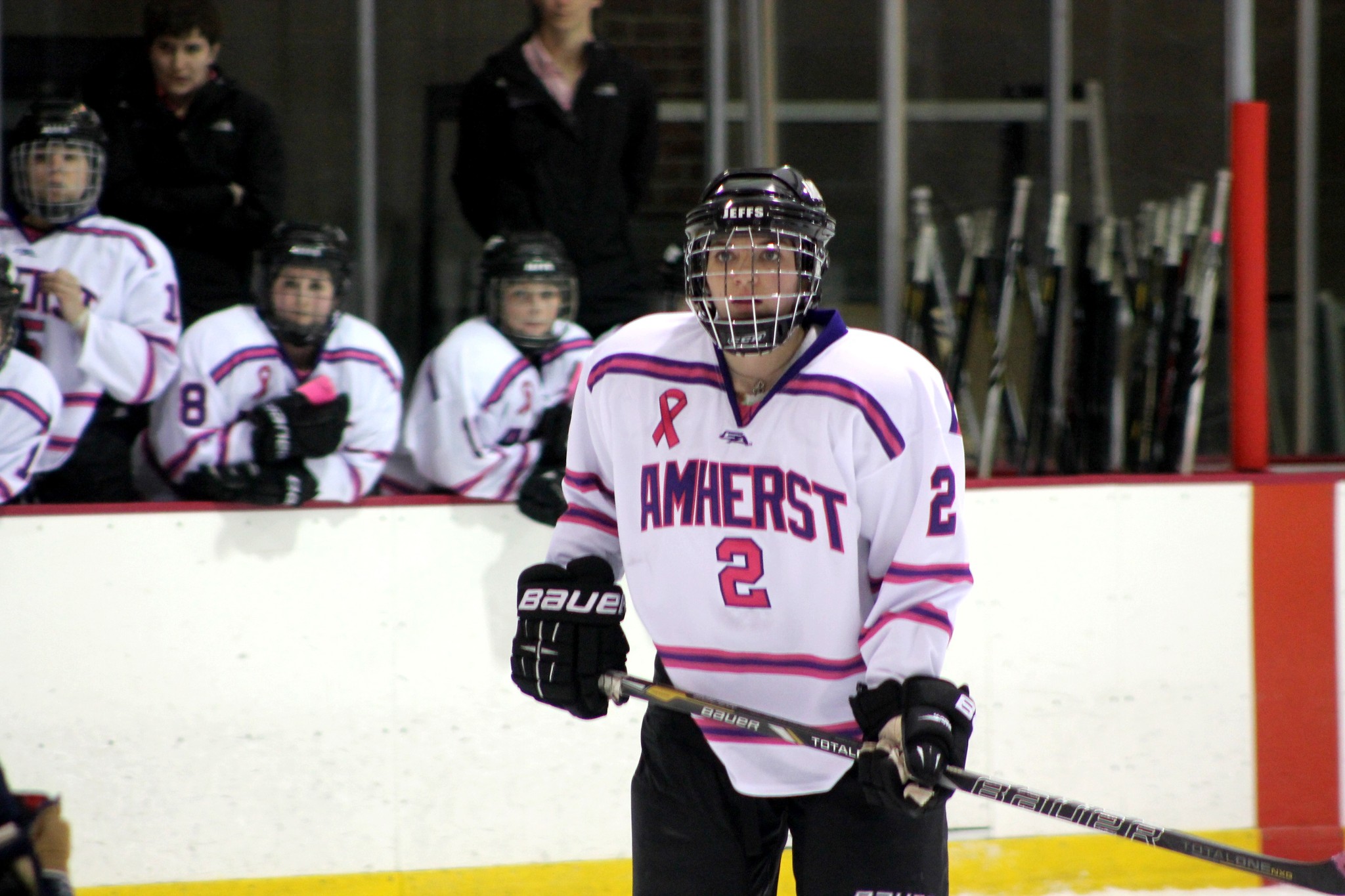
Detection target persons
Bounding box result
[450,0,660,340]
[0,102,182,505]
[379,230,594,502]
[510,160,976,896]
[98,0,285,324]
[129,215,404,506]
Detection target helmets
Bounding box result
[684,166,836,357]
[482,234,577,349]
[9,100,107,225]
[248,218,357,345]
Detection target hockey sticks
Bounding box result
[888,170,1232,479]
[591,670,1345,896]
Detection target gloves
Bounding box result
[245,465,317,510]
[848,675,975,812]
[527,403,572,475]
[510,479,630,720]
[518,467,568,528]
[236,391,348,461]
